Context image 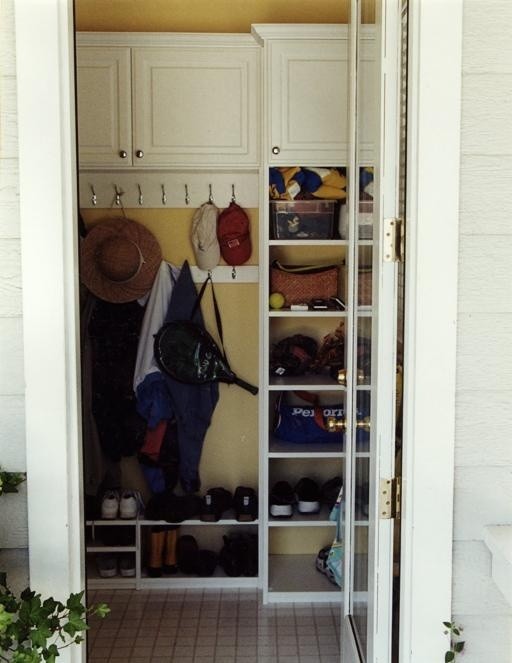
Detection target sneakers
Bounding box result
[199,487,231,521]
[97,558,117,577]
[270,482,295,518]
[102,488,119,519]
[118,554,135,576]
[296,478,320,514]
[234,486,257,521]
[121,489,143,519]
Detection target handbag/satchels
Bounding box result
[272,404,370,443]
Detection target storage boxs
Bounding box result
[269,198,338,239]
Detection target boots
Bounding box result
[149,528,179,576]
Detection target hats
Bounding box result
[216,203,251,266]
[81,216,162,303]
[192,203,221,270]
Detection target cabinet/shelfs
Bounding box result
[261,165,373,604]
[266,37,375,165]
[76,29,261,172]
[85,517,260,591]
